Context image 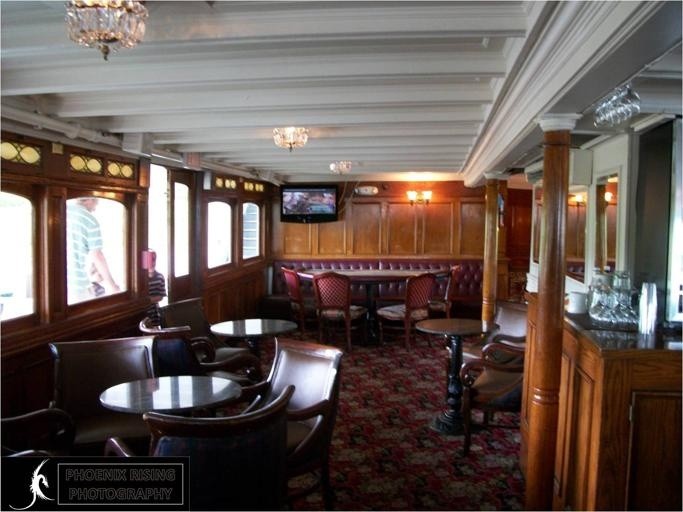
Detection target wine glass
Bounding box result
[592,83,643,127]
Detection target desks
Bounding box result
[413,318,493,433]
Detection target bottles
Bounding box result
[593,267,601,273]
[603,265,612,273]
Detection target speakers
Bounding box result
[122,133,154,157]
[569,149,593,192]
[203,171,212,190]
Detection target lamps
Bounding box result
[329,160,354,174]
[62,0,147,64]
[405,188,433,207]
[270,124,309,154]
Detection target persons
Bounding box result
[67,197,120,299]
[147,248,167,328]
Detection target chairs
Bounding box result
[266,255,490,351]
[462,300,531,357]
[1,292,354,511]
[450,334,529,454]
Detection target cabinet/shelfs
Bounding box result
[522,289,681,510]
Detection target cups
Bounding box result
[564,291,588,314]
[637,279,658,336]
[587,271,638,324]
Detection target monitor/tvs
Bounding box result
[280,184,338,223]
